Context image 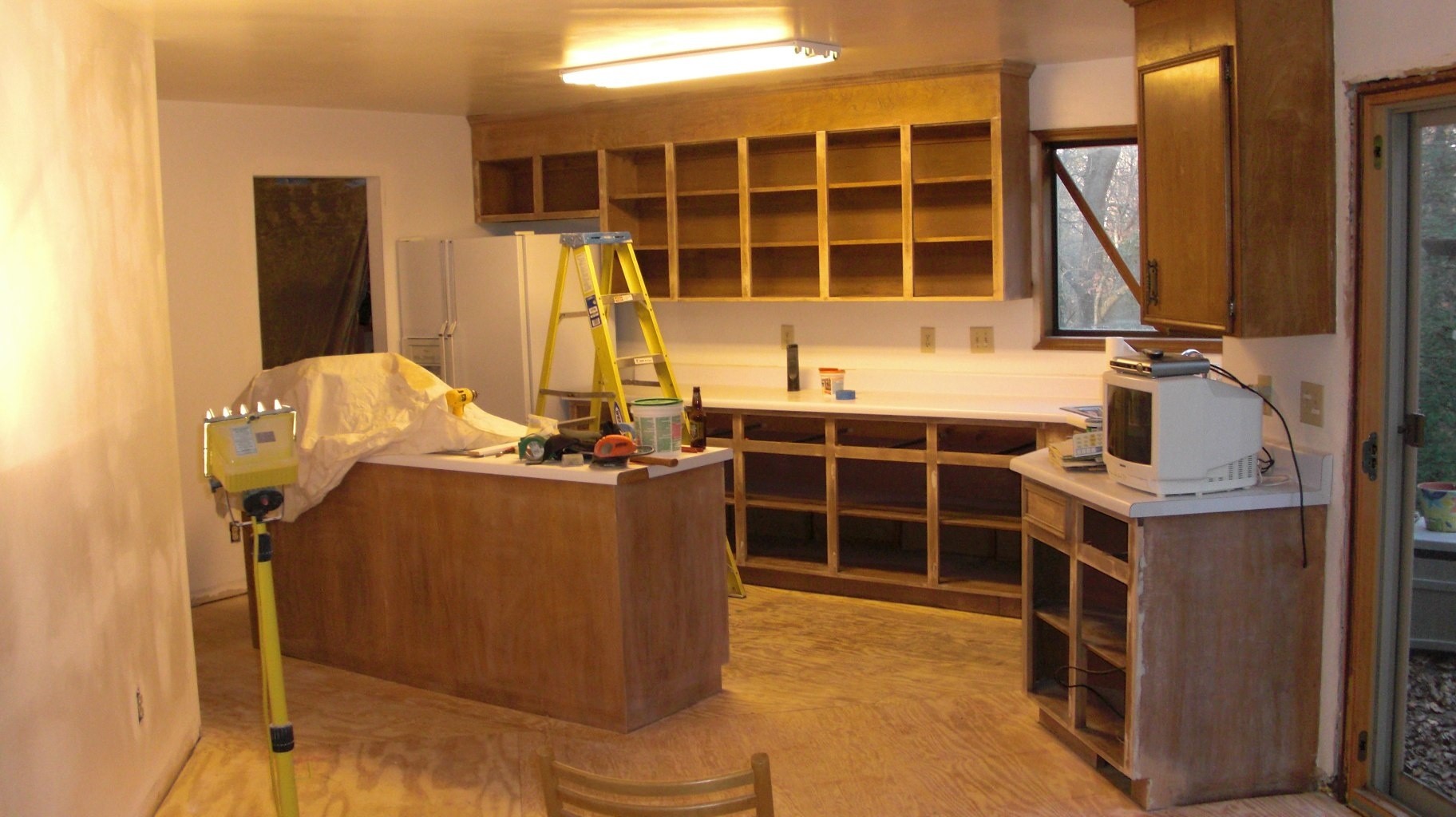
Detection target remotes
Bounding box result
[1142,348,1163,360]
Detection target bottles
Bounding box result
[689,386,707,452]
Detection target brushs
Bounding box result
[496,445,515,458]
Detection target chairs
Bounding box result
[536,744,774,817]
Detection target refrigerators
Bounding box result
[396,224,617,442]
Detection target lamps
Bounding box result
[557,39,842,89]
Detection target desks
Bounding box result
[239,427,732,734]
[560,361,1337,814]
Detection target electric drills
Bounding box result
[447,389,480,419]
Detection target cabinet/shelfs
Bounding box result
[1019,476,1139,780]
[465,57,1036,304]
[1123,0,1338,339]
[572,405,1044,600]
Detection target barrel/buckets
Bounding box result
[820,366,845,397]
[630,397,684,459]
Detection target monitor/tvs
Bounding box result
[1101,370,1263,498]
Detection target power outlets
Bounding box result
[921,326,936,354]
[780,323,795,350]
[969,325,995,354]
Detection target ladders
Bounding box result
[533,232,747,600]
